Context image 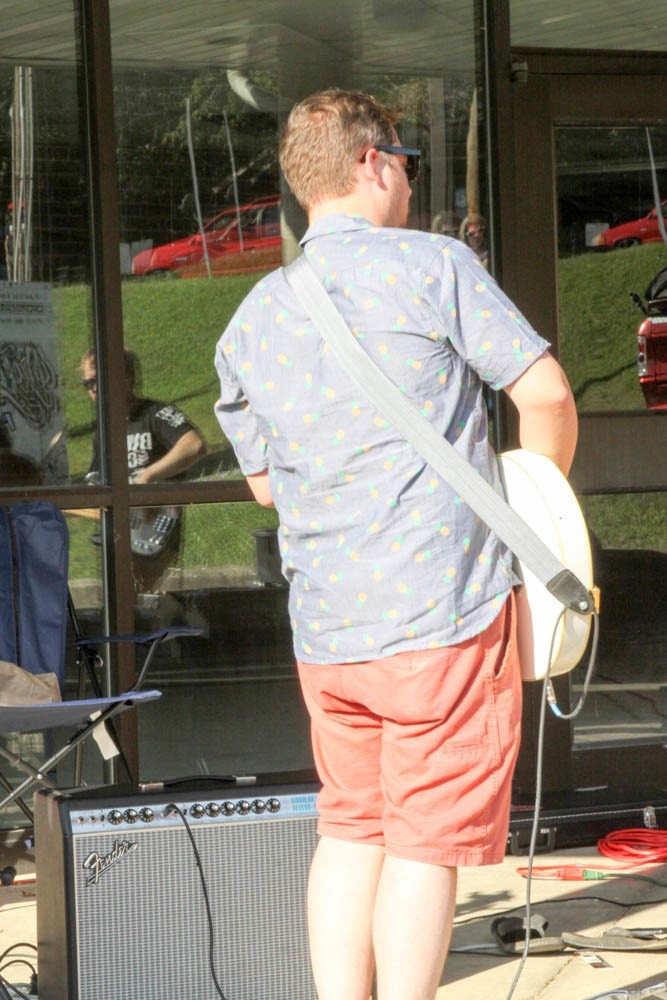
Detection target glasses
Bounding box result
[465,227,485,237]
[360,144,421,183]
[83,379,97,390]
[441,229,459,236]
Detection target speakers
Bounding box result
[33,768,318,1000]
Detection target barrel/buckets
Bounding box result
[250,530,284,583]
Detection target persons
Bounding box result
[431,211,492,272]
[164,192,199,241]
[52,348,208,606]
[0,411,69,706]
[213,91,579,1000]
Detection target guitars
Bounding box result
[61,505,184,562]
[496,445,595,684]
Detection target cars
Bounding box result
[132,194,282,279]
[638,266,667,411]
[592,200,667,252]
